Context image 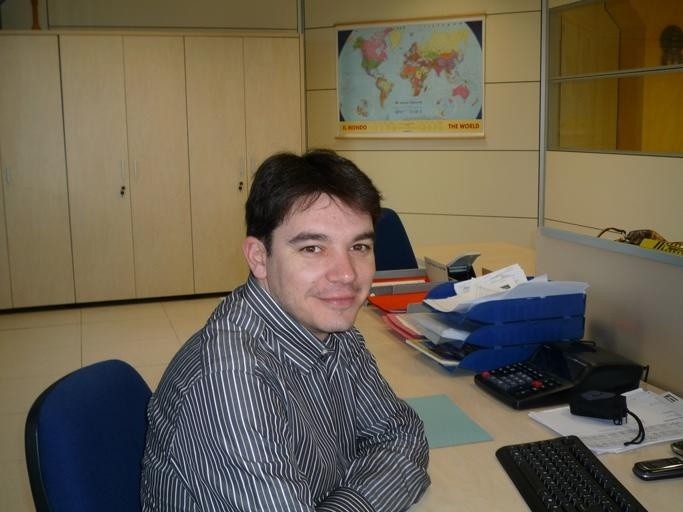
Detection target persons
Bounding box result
[135,146,433,511]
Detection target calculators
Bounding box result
[473,342,643,409]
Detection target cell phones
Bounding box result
[632,457,683,481]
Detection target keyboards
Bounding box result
[496,435,648,512]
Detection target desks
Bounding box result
[426,239,558,291]
[320,304,682,512]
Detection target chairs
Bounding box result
[23,358,148,512]
[358,207,418,269]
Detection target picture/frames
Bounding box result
[333,16,488,135]
